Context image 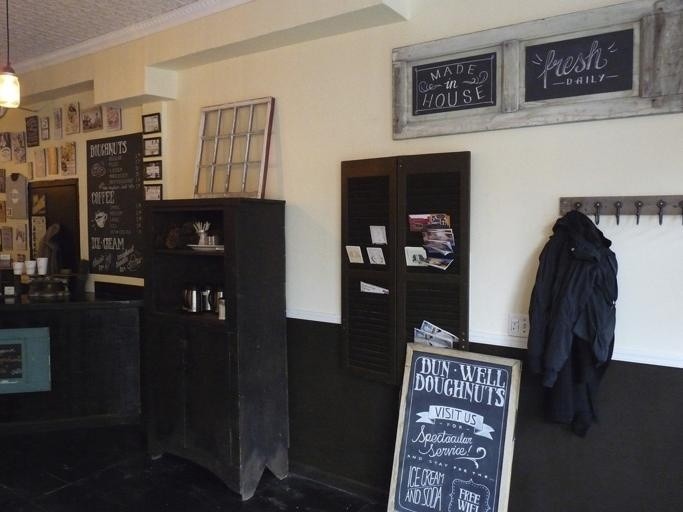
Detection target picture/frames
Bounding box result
[141,112,163,201]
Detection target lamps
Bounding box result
[0,0,22,110]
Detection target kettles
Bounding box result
[182,284,224,313]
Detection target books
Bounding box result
[402,212,455,271]
[368,225,386,246]
[345,245,364,263]
[419,319,459,343]
[412,327,453,349]
[365,247,386,265]
[359,280,389,295]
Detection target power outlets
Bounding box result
[507,312,531,338]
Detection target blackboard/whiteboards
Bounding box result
[86,133,145,289]
[387,344,522,512]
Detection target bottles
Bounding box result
[218,298,225,320]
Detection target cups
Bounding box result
[12,257,49,275]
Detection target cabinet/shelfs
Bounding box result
[140,197,291,502]
